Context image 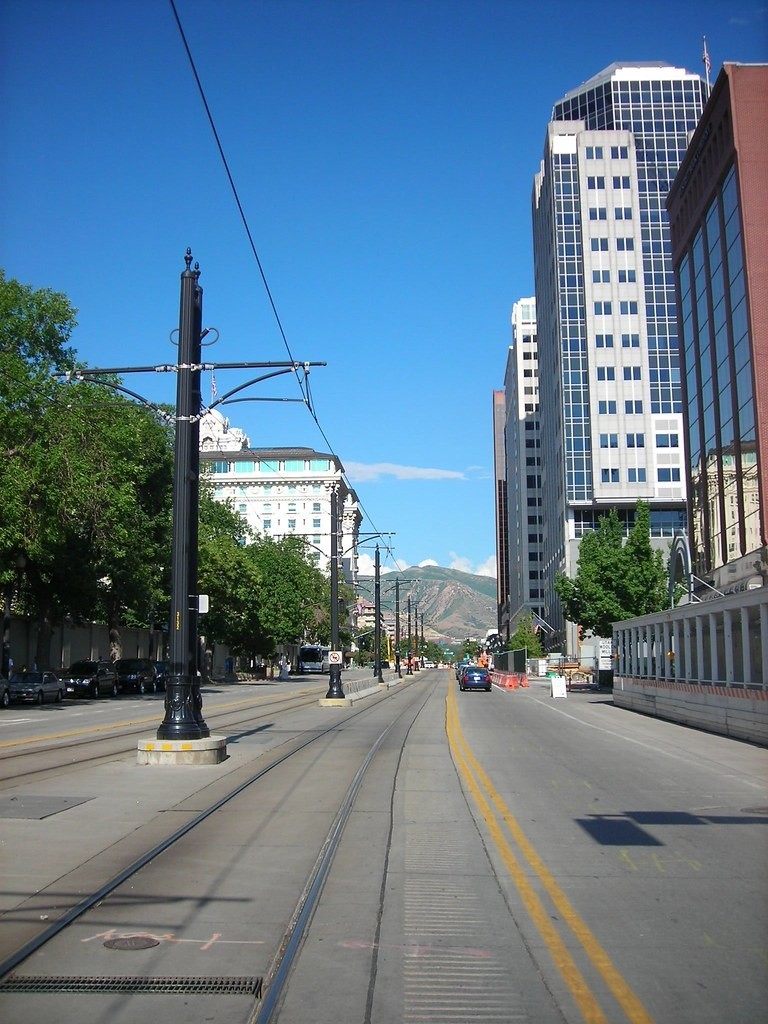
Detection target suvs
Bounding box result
[113,659,166,694]
[58,660,119,699]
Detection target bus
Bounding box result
[299,645,343,674]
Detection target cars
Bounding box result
[9,671,66,705]
[0,673,11,708]
[453,660,492,692]
[425,660,435,668]
